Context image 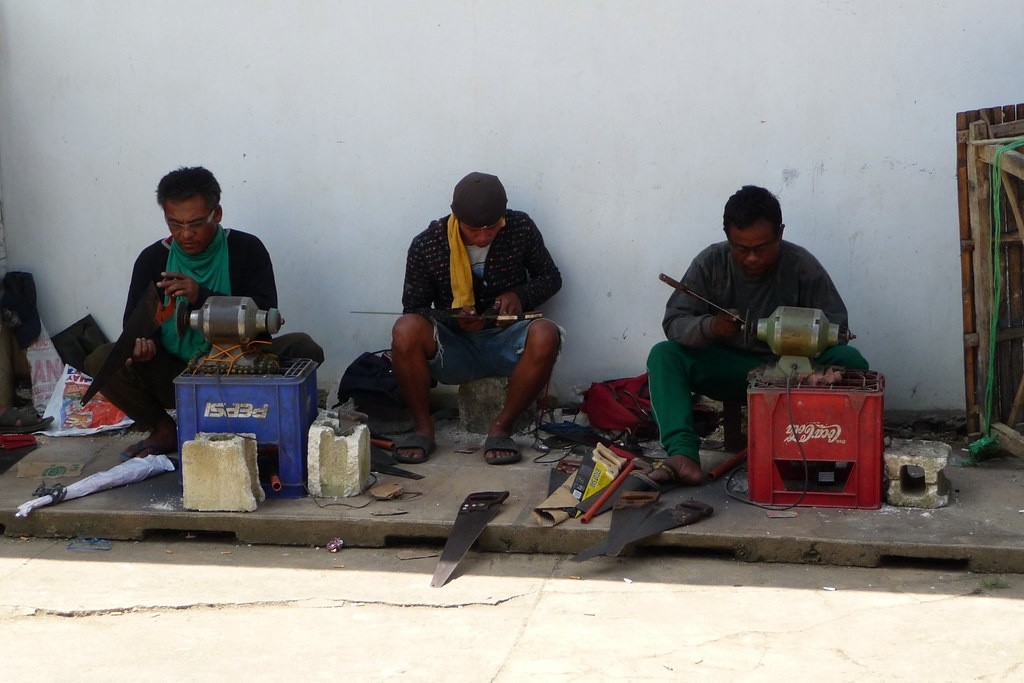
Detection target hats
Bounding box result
[451,172,508,228]
[0,271,41,349]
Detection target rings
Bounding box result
[494,300,500,304]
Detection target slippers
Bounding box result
[120,441,178,461]
[632,463,701,492]
[484,437,522,464]
[392,436,436,464]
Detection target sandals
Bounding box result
[0,407,54,434]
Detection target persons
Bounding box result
[631,186,868,483]
[392,172,563,463]
[0,321,54,434]
[84,166,278,461]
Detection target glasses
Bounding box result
[730,230,779,254]
[165,209,215,231]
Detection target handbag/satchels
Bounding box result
[332,349,407,420]
[581,371,652,430]
